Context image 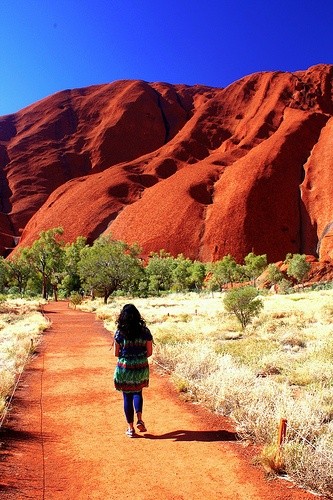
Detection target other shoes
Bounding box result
[136,420,147,432]
[126,430,136,438]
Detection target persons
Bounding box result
[112,303,154,437]
